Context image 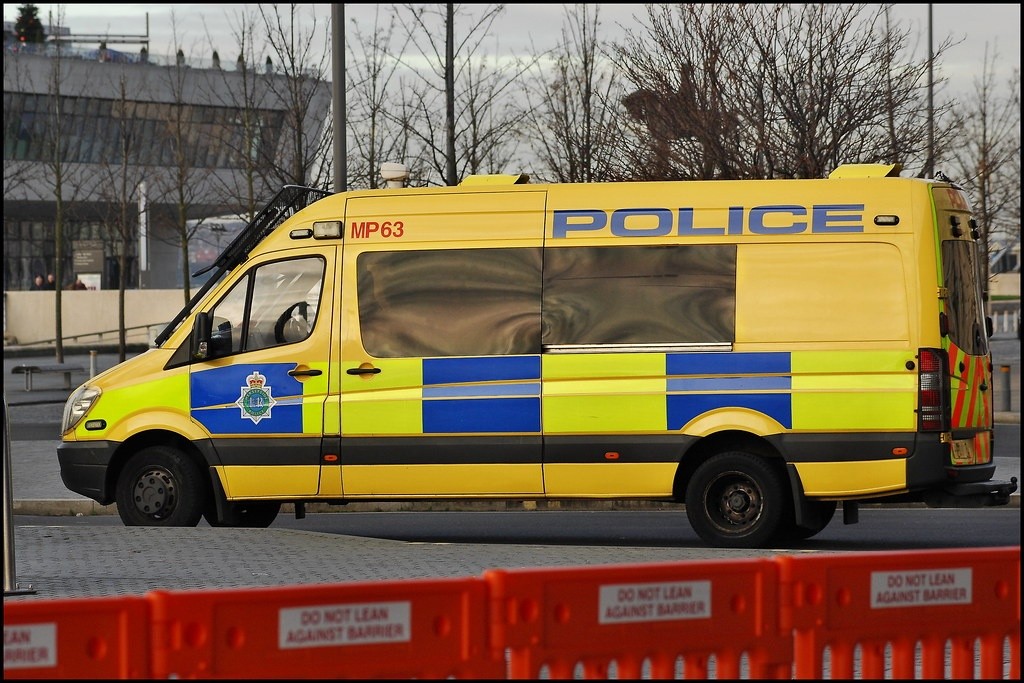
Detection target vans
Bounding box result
[53,162,1018,547]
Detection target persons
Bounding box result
[72,279,88,290]
[29,272,56,291]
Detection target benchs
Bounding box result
[12,362,85,391]
